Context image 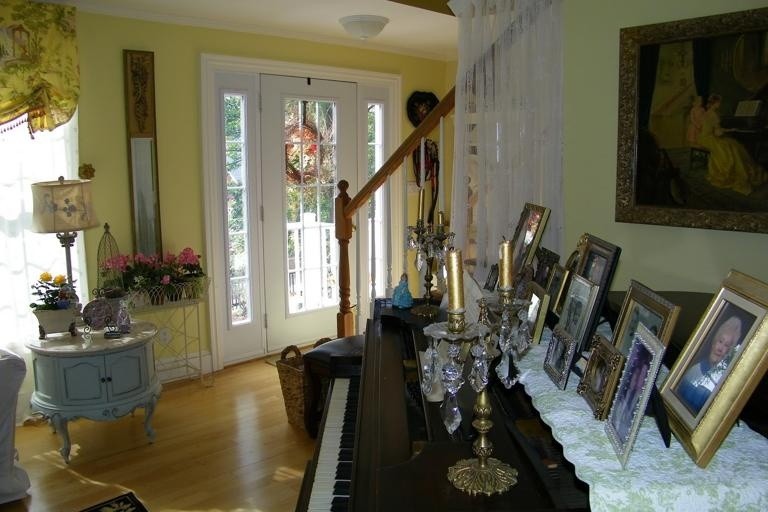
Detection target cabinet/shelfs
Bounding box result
[25,319,164,464]
[92,279,215,388]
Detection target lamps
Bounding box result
[340,15,388,43]
[31,176,98,287]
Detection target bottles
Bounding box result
[117,306,131,333]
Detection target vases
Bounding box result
[102,273,206,307]
[32,303,82,339]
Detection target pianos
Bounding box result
[296,290,767,511]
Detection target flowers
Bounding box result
[101,248,203,287]
[30,272,77,309]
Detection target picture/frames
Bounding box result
[615,6,768,234]
[660,268,768,470]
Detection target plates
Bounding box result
[83,300,112,328]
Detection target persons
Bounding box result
[675,317,742,416]
[392,273,414,310]
[687,94,764,196]
[612,361,649,445]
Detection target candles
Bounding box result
[446,247,464,312]
[420,137,424,188]
[439,116,445,212]
